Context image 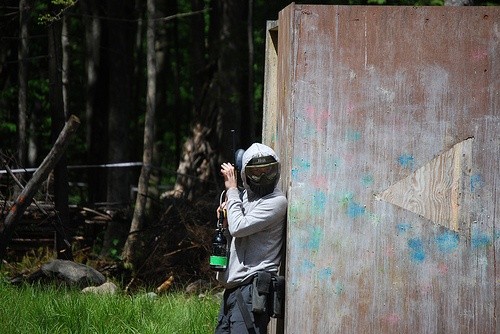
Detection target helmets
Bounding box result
[244,156,282,195]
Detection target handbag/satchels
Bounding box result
[252,270,283,318]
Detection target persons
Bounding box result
[216,142,287,334]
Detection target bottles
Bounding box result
[209,228,227,271]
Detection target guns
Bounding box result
[210,129,245,271]
